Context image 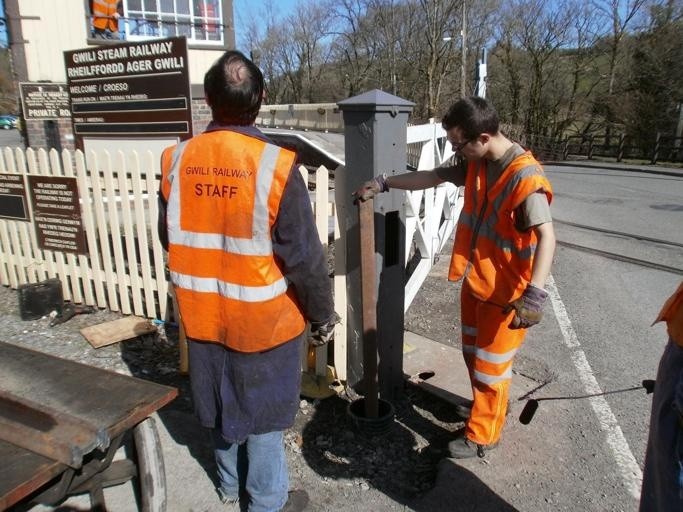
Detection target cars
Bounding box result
[0,115,21,130]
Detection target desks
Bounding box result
[0,340,178,512]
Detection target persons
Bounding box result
[87,0,125,39]
[638,281,683,507]
[351,95,558,460]
[155,45,343,512]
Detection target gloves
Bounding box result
[310,321,335,347]
[503,283,548,330]
[351,174,390,201]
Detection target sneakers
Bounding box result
[277,490,308,512]
[448,436,499,459]
[95,31,121,40]
[457,399,511,417]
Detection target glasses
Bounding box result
[452,137,473,152]
